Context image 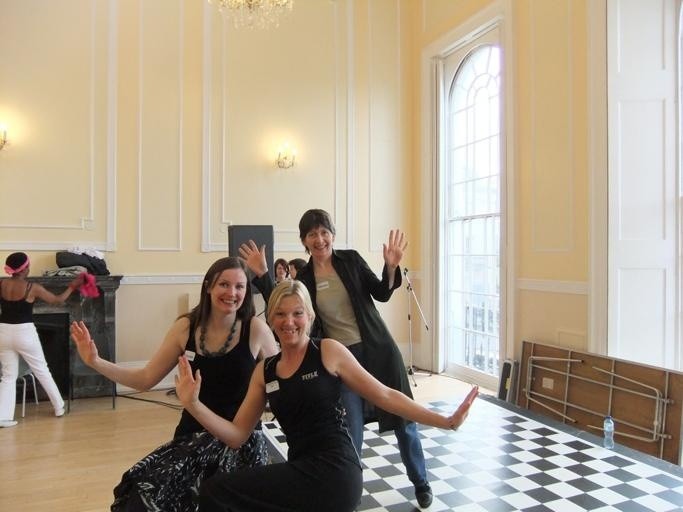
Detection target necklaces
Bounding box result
[198,319,239,358]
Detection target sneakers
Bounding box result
[55,409,64,416]
[0,420,18,427]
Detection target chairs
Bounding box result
[68,328,117,412]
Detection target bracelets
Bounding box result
[69,283,76,289]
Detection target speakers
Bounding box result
[228,225,274,293]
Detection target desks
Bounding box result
[517,340,682,465]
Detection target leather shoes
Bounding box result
[415,481,433,509]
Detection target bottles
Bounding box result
[603,414,615,450]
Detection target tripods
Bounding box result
[403,268,432,386]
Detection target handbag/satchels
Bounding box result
[43,251,110,277]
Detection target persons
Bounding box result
[67,258,280,512]
[174,278,480,511]
[0,250,90,428]
